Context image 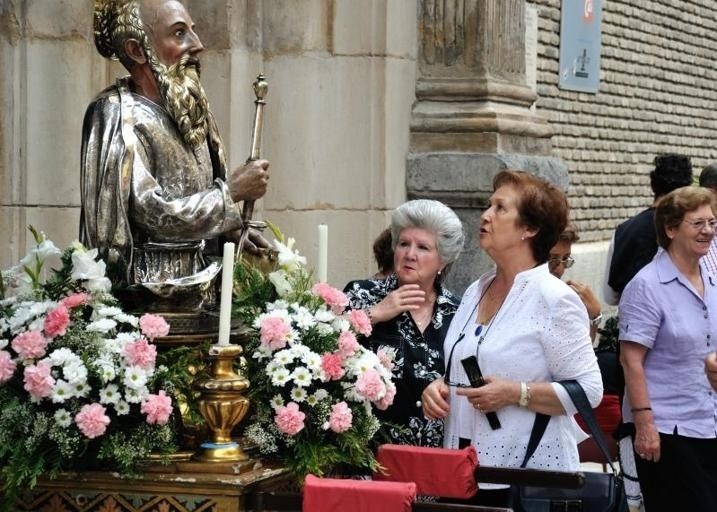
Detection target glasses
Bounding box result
[549,256,574,270]
[444,334,484,387]
[682,219,717,230]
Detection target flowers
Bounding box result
[0,225,178,512]
[238,221,397,481]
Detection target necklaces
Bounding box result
[475,285,502,336]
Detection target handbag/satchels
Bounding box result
[596,350,624,396]
[371,376,433,423]
[514,467,628,512]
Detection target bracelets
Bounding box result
[365,308,375,326]
[631,407,652,412]
[519,382,530,408]
[589,311,603,326]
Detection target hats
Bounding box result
[649,154,692,192]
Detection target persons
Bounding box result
[615,186,717,512]
[363,224,395,279]
[700,163,717,282]
[79,2,271,309]
[421,168,603,506]
[339,198,473,503]
[546,222,603,350]
[607,154,694,305]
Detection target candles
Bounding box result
[318,223,329,283]
[218,242,235,345]
